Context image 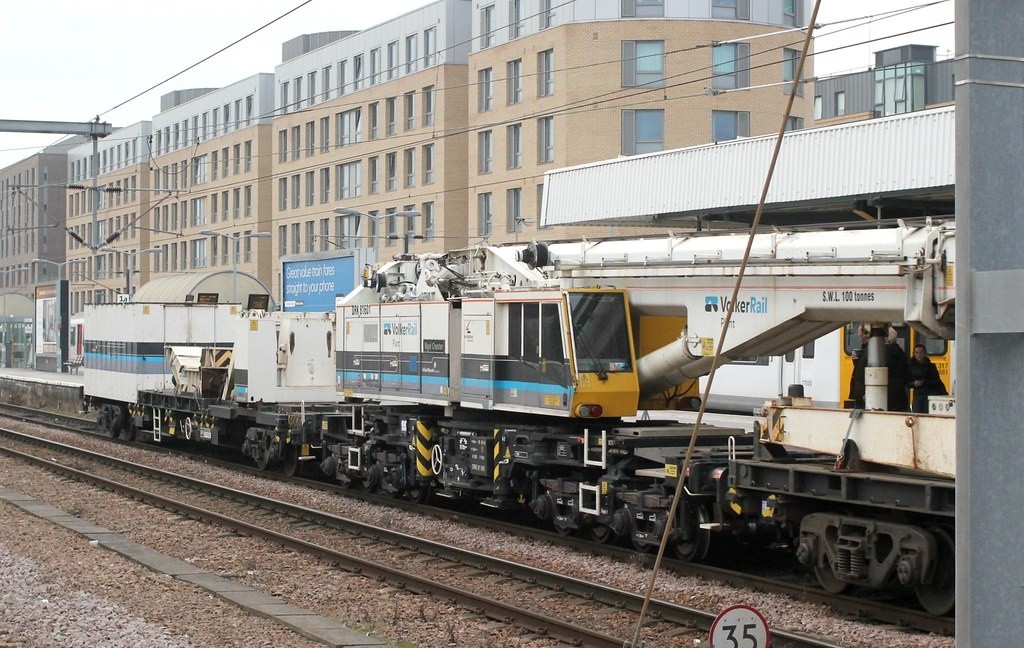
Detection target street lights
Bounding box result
[31,258,87,288]
[332,207,424,267]
[197,230,272,306]
[99,247,164,301]
[0,267,30,367]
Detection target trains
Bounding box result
[68,215,957,631]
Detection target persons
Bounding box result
[849,323,948,414]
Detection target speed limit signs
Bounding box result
[709,605,770,648]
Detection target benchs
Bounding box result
[63,354,84,375]
